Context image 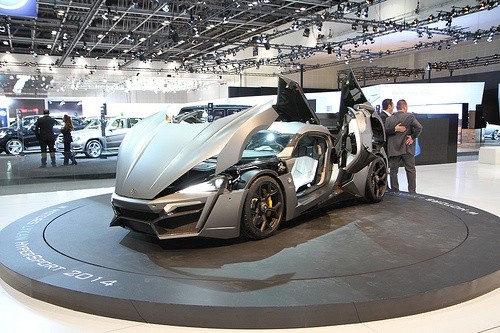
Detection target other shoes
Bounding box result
[70,162,77,165]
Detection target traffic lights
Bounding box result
[100,104,107,116]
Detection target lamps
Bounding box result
[101,0,500,74]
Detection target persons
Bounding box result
[58,115,78,167]
[32,109,62,171]
[375,98,423,195]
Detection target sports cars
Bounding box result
[109,69,390,241]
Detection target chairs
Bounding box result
[291,141,326,193]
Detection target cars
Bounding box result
[0,115,87,157]
[458,118,500,140]
[55,117,144,159]
